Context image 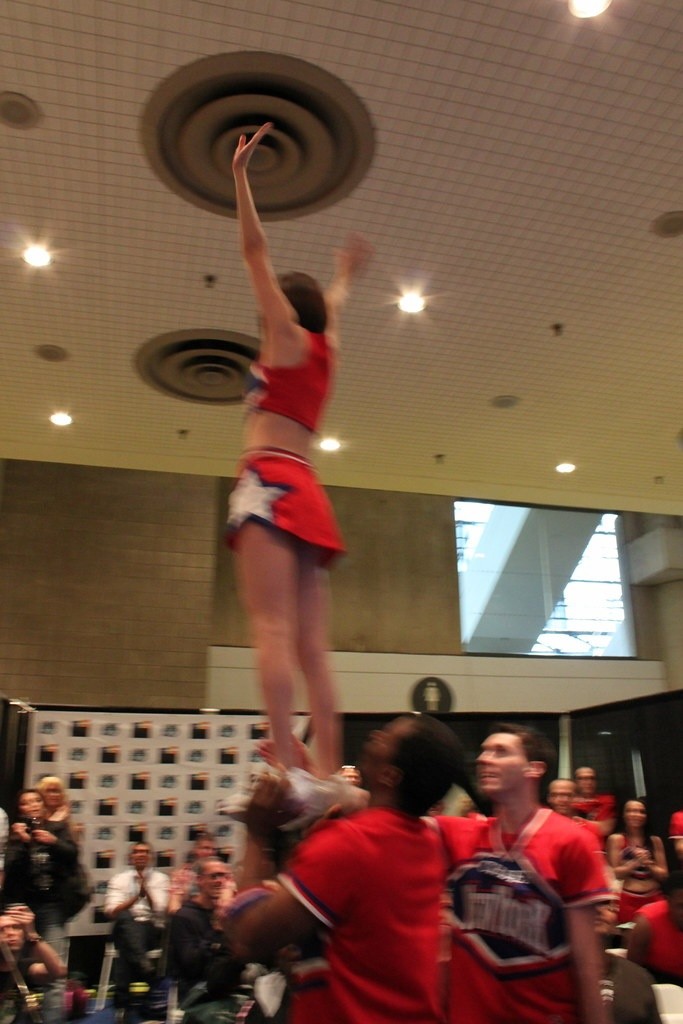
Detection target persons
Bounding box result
[227,123,367,778]
[0,710,683,1024]
[607,799,668,948]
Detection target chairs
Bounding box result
[94,940,171,1011]
[604,948,683,1024]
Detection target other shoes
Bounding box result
[218,765,369,831]
[137,958,156,983]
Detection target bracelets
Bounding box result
[30,936,42,946]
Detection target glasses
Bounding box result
[594,899,620,911]
[201,872,229,879]
